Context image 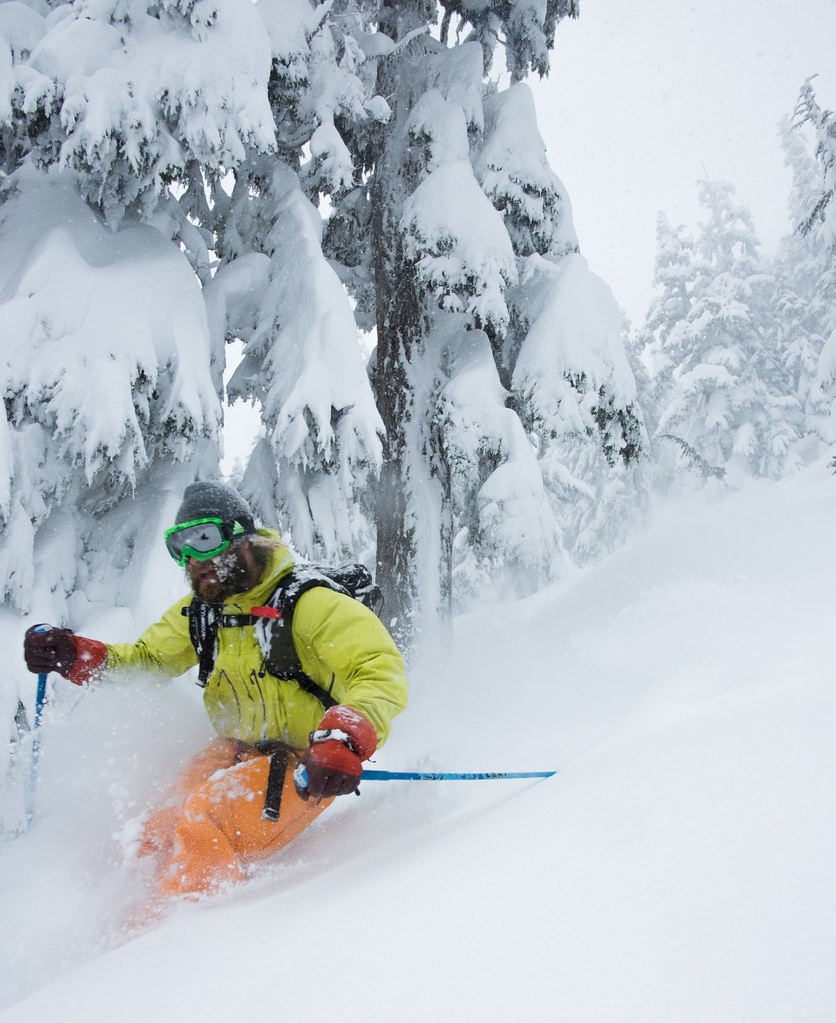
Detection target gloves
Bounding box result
[23,623,108,686]
[296,704,377,801]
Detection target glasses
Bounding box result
[165,516,256,567]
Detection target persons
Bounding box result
[24,480,407,948]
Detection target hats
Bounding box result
[176,481,250,524]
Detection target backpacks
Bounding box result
[189,558,385,710]
